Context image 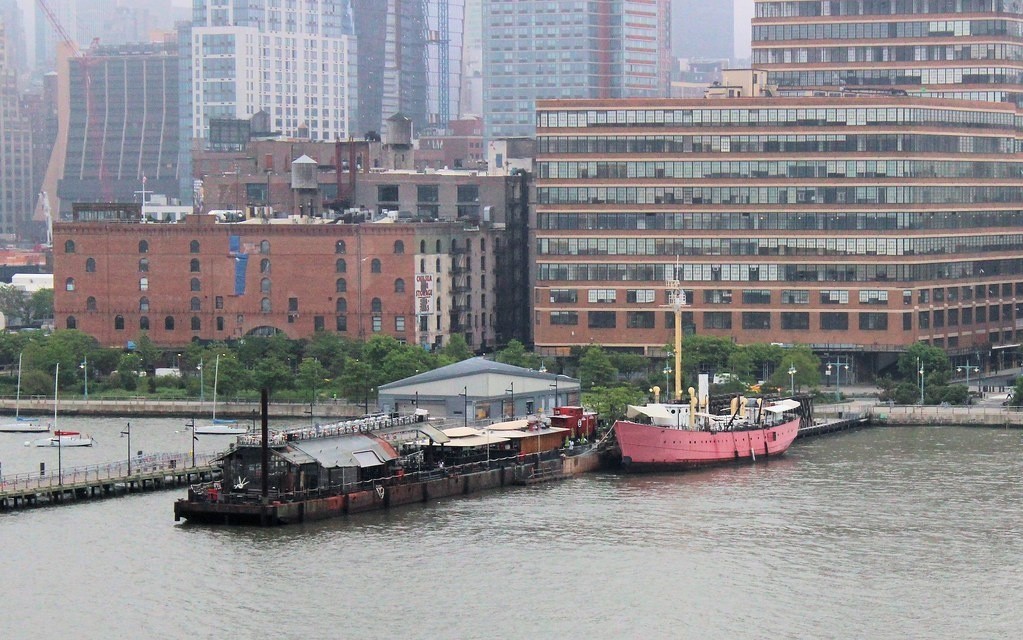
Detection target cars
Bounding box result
[750,383,783,392]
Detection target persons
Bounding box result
[439,460,444,469]
[568,439,574,450]
[1006,388,1011,399]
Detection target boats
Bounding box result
[614,256,802,465]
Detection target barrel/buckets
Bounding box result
[208,489,217,500]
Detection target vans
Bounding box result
[713,372,739,384]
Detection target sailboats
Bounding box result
[0,352,49,432]
[32,362,92,446]
[195,354,247,435]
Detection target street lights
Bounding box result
[185,425,195,470]
[787,367,796,396]
[304,411,313,426]
[49,438,61,487]
[79,362,88,401]
[411,399,418,409]
[549,385,557,407]
[504,390,513,421]
[662,366,672,403]
[365,386,374,414]
[196,363,203,403]
[956,366,979,386]
[919,368,924,404]
[591,381,599,413]
[827,363,849,402]
[248,414,256,437]
[458,393,467,427]
[358,405,367,414]
[121,432,130,474]
